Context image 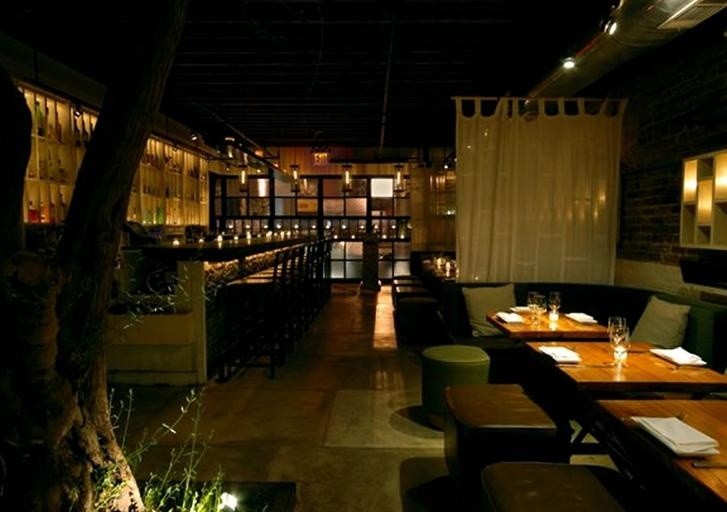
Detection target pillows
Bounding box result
[630,293,693,349]
[459,282,519,339]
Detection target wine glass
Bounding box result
[528,291,562,328]
[607,317,631,369]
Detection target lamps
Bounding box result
[239,151,251,193]
[392,158,405,193]
[340,160,355,192]
[596,15,620,36]
[288,146,302,193]
[556,48,577,71]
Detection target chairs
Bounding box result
[216,235,334,384]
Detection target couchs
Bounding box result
[439,281,727,402]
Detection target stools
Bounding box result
[420,343,618,512]
[393,278,442,347]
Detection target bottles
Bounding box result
[27,94,211,230]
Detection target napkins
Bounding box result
[564,313,598,324]
[648,346,708,366]
[496,312,525,324]
[538,346,582,363]
[629,416,720,455]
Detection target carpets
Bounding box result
[324,388,454,450]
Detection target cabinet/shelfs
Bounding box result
[677,148,727,249]
